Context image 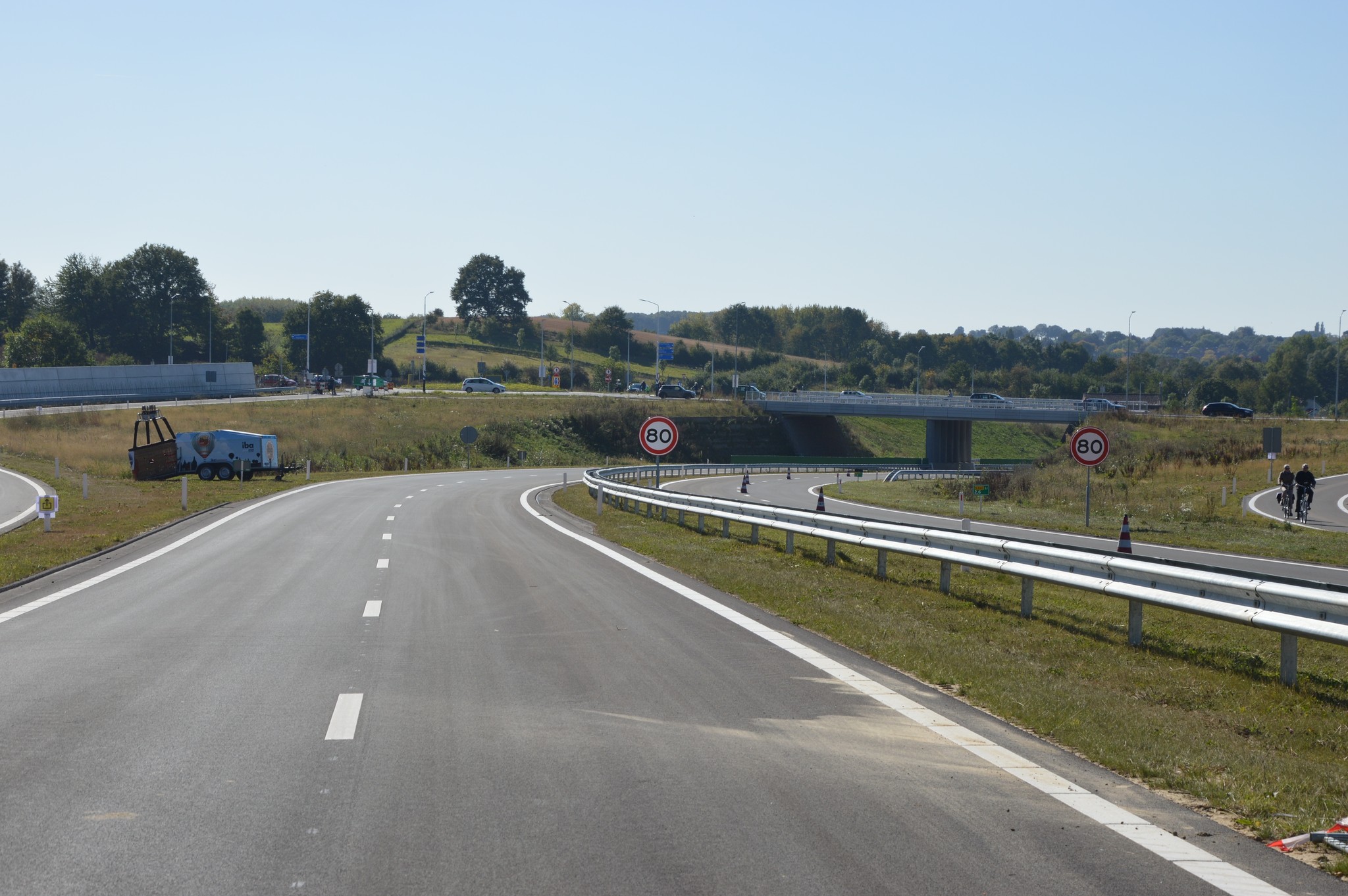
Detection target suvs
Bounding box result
[838,390,874,404]
[969,393,1015,409]
[462,377,507,395]
[1201,401,1255,418]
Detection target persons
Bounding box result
[615,380,621,393]
[691,382,698,394]
[698,386,705,400]
[640,381,646,393]
[280,375,285,387]
[292,375,298,383]
[789,386,797,393]
[658,381,661,387]
[1294,464,1316,520]
[1115,401,1119,410]
[660,381,665,387]
[645,386,649,394]
[1277,464,1295,516]
[1082,397,1088,411]
[947,390,953,397]
[328,375,336,395]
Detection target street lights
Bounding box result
[169,293,182,365]
[971,363,977,395]
[1312,309,1348,419]
[916,346,926,407]
[1138,382,1145,411]
[710,302,746,401]
[1126,310,1136,410]
[306,292,434,393]
[540,300,574,392]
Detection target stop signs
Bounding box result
[604,376,611,383]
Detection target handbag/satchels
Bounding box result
[1276,492,1282,504]
[1292,494,1295,503]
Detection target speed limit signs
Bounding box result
[1070,425,1110,466]
[637,417,679,456]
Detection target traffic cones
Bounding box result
[740,467,792,494]
[1116,512,1133,554]
[815,487,825,512]
[847,466,851,477]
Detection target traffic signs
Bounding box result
[291,334,308,339]
[658,342,674,361]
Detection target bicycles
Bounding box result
[1276,483,1313,525]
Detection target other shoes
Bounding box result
[1308,506,1311,509]
[1290,512,1293,516]
[1296,517,1299,519]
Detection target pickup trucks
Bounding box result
[1073,398,1126,411]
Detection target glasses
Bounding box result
[1303,467,1308,469]
[1285,468,1290,470]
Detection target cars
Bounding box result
[627,383,767,400]
[260,375,296,391]
